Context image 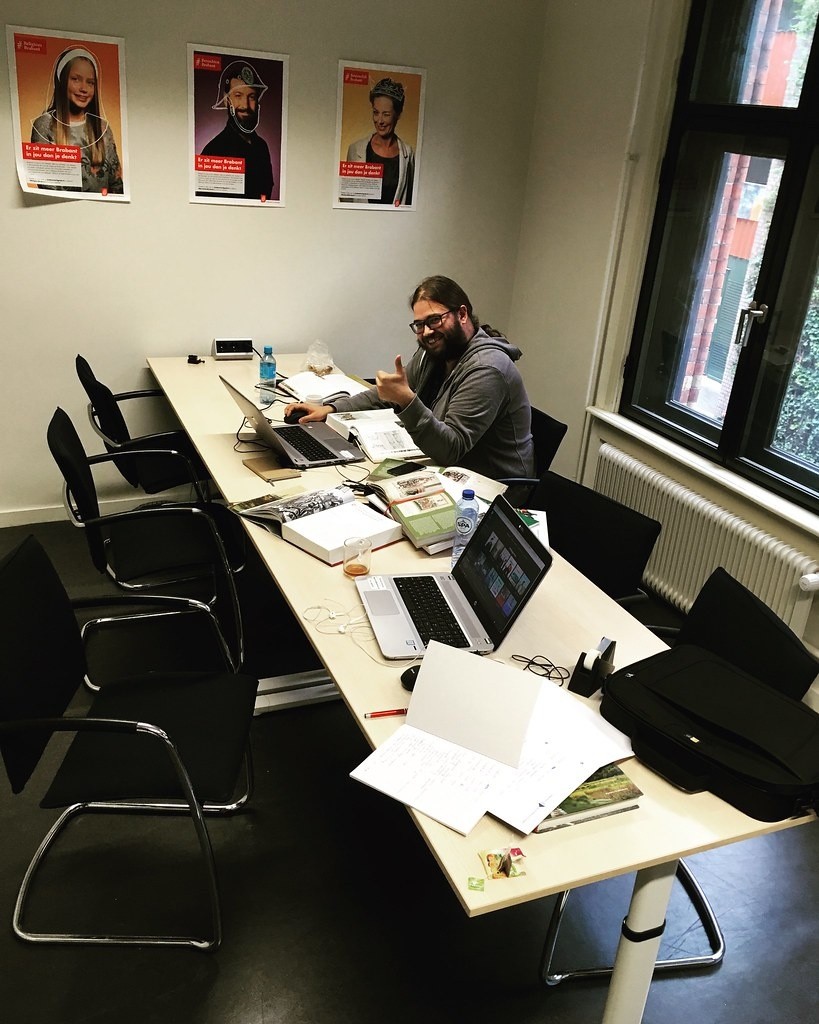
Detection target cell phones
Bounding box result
[387,462,427,477]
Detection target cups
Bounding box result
[343,536,373,578]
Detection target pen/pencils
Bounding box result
[365,708,408,718]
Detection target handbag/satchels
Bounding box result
[600,646,819,822]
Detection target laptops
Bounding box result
[356,495,554,660]
[219,375,365,469]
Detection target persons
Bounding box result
[517,581,525,593]
[285,276,534,478]
[30,48,123,193]
[196,63,272,200]
[486,538,493,551]
[497,848,526,877]
[340,78,414,205]
[504,564,512,576]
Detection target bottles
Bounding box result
[450,489,479,570]
[305,394,323,406]
[260,345,276,405]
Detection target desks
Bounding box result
[146,354,819,1024]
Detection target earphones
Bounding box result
[330,612,345,618]
[339,624,346,634]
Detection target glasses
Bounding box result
[409,310,452,334]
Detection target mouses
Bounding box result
[284,409,309,424]
[401,665,421,691]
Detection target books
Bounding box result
[278,371,370,403]
[365,458,539,554]
[350,639,644,836]
[325,408,426,462]
[227,485,409,567]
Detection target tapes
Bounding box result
[583,638,612,671]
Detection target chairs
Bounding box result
[0,354,819,986]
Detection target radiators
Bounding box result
[593,441,819,642]
